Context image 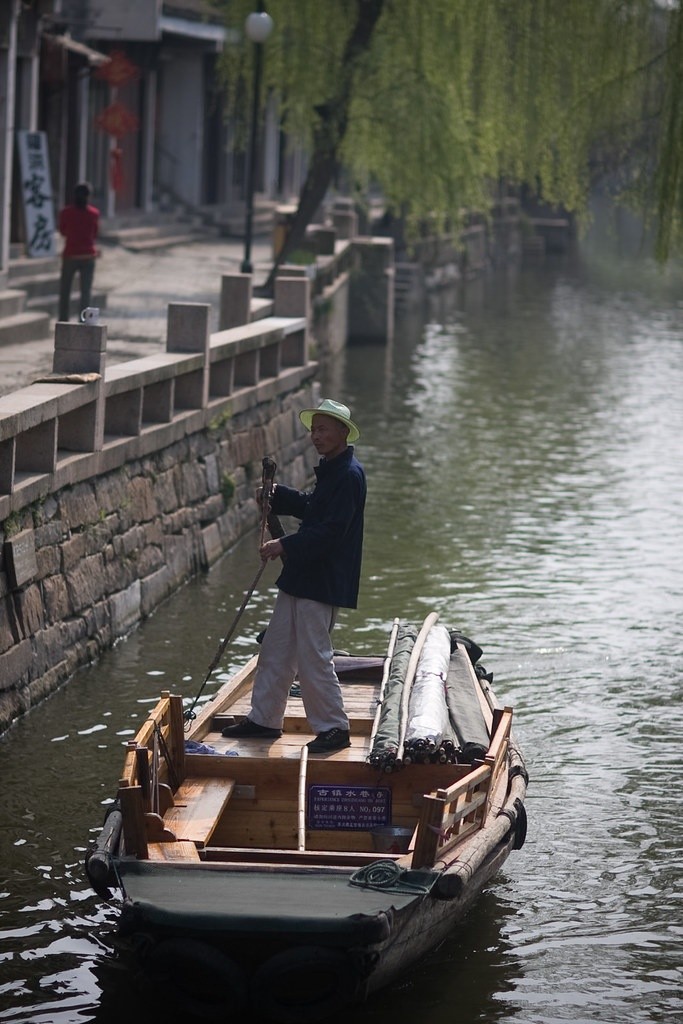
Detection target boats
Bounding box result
[86,612,530,1004]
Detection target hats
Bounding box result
[299,398,360,444]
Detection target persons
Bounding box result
[57,183,99,326]
[219,399,366,752]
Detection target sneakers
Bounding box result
[306,728,354,755]
[221,717,284,739]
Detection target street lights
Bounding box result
[241,0,276,273]
